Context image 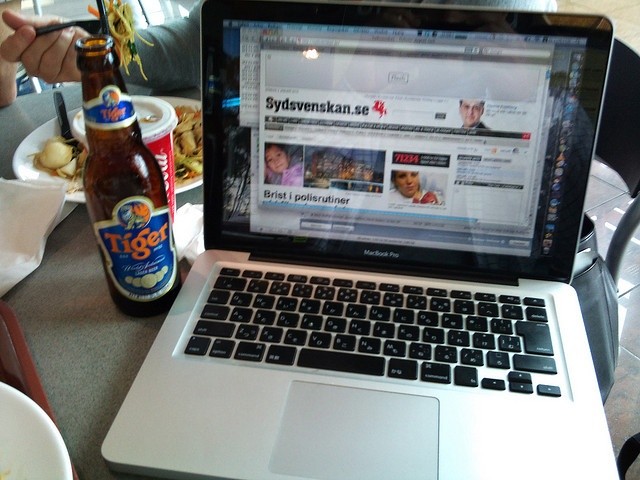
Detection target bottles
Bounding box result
[73,33,182,318]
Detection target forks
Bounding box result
[34,7,126,38]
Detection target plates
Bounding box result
[11,95,203,204]
[0,381,73,480]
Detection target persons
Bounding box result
[389,169,444,206]
[0,0,557,88]
[265,143,302,186]
[458,99,488,129]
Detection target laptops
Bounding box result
[101,1,620,480]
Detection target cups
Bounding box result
[70,94,179,226]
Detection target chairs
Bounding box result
[593,36,640,288]
